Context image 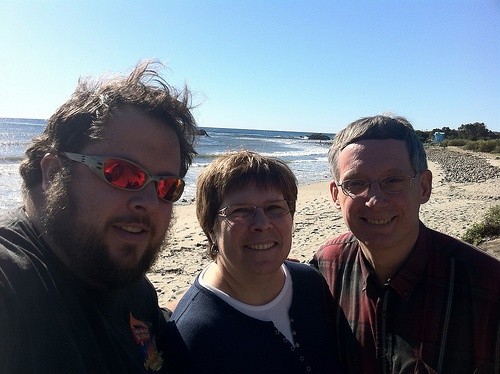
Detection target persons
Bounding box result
[0,58,197,374]
[166,151,361,373]
[300,114,500,373]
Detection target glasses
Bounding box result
[336,171,421,195]
[217,200,293,220]
[58,152,186,202]
